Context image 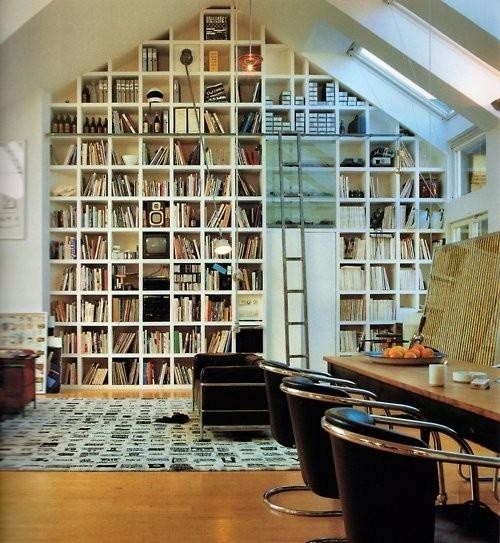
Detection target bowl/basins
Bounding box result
[122,155,138,164]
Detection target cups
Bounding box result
[429,364,447,387]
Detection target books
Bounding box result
[203,110,225,134]
[400,267,416,291]
[176,202,190,227]
[205,267,220,291]
[112,359,139,385]
[205,203,231,227]
[205,147,213,165]
[400,237,416,260]
[54,329,77,354]
[419,239,445,260]
[58,358,76,384]
[142,46,157,72]
[51,295,77,322]
[142,142,168,165]
[174,264,201,290]
[112,109,139,134]
[174,327,201,353]
[418,268,425,290]
[50,171,77,196]
[83,362,108,384]
[205,330,232,353]
[144,361,170,384]
[340,235,365,260]
[174,362,193,384]
[237,173,258,196]
[236,203,261,227]
[174,172,200,196]
[370,175,379,198]
[113,331,139,353]
[143,176,169,195]
[206,15,228,40]
[340,324,365,351]
[82,139,106,165]
[50,200,78,228]
[370,234,395,260]
[205,295,232,321]
[83,204,107,228]
[381,206,394,230]
[341,266,365,290]
[113,298,139,322]
[164,207,169,226]
[86,78,108,103]
[113,205,135,227]
[51,140,76,166]
[371,324,396,351]
[339,206,366,232]
[113,149,124,165]
[82,172,107,196]
[404,205,417,229]
[81,265,108,291]
[115,79,139,103]
[82,297,108,322]
[238,143,260,165]
[400,142,415,167]
[241,111,261,133]
[205,172,231,196]
[82,234,106,259]
[240,268,262,290]
[52,264,75,291]
[371,298,396,321]
[205,234,231,259]
[339,174,349,199]
[144,330,169,353]
[174,296,201,321]
[238,236,262,259]
[370,266,391,290]
[175,139,199,165]
[112,173,137,196]
[398,176,415,198]
[49,234,77,260]
[113,265,126,290]
[173,235,200,259]
[253,82,261,102]
[82,329,108,354]
[340,298,366,322]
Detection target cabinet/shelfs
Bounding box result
[41,4,448,388]
[1,348,44,418]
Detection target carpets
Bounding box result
[0,398,303,473]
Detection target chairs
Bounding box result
[263,375,448,516]
[306,407,500,541]
[255,359,356,448]
[458,440,500,503]
[191,354,271,441]
